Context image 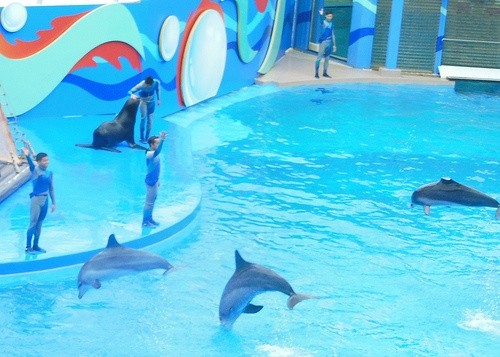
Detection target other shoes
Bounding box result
[315,76,319,78]
[33,246,46,253]
[145,138,149,141]
[25,247,36,254]
[140,139,147,144]
[150,220,158,225]
[142,222,156,227]
[323,73,331,78]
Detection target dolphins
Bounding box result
[218,248,315,329]
[410,177,500,220]
[77,234,177,299]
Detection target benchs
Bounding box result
[438,65,500,92]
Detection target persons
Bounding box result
[128,76,160,144]
[22,147,55,254]
[142,132,168,228]
[314,8,336,78]
